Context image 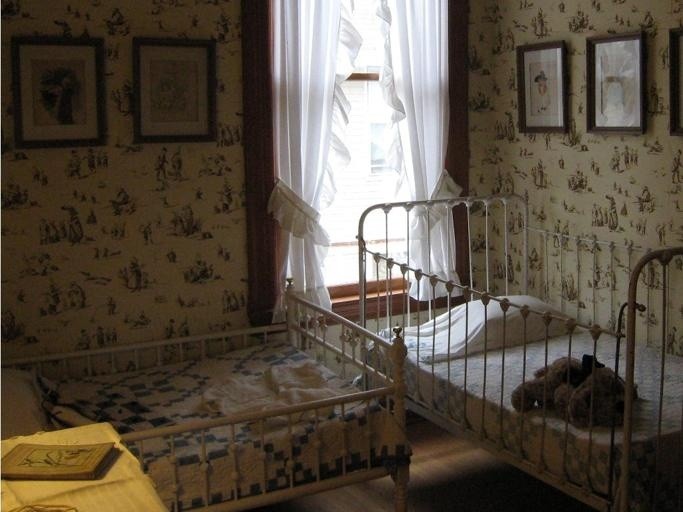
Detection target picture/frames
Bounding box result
[132,35,218,144]
[669,26,683,136]
[516,40,569,134]
[12,33,109,148]
[585,31,646,135]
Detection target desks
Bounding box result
[0,420,170,512]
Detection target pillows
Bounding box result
[406,294,573,361]
[1,365,52,437]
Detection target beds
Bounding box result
[1,278,409,512]
[359,194,683,512]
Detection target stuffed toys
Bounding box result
[511,354,638,429]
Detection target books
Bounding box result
[1,442,119,480]
